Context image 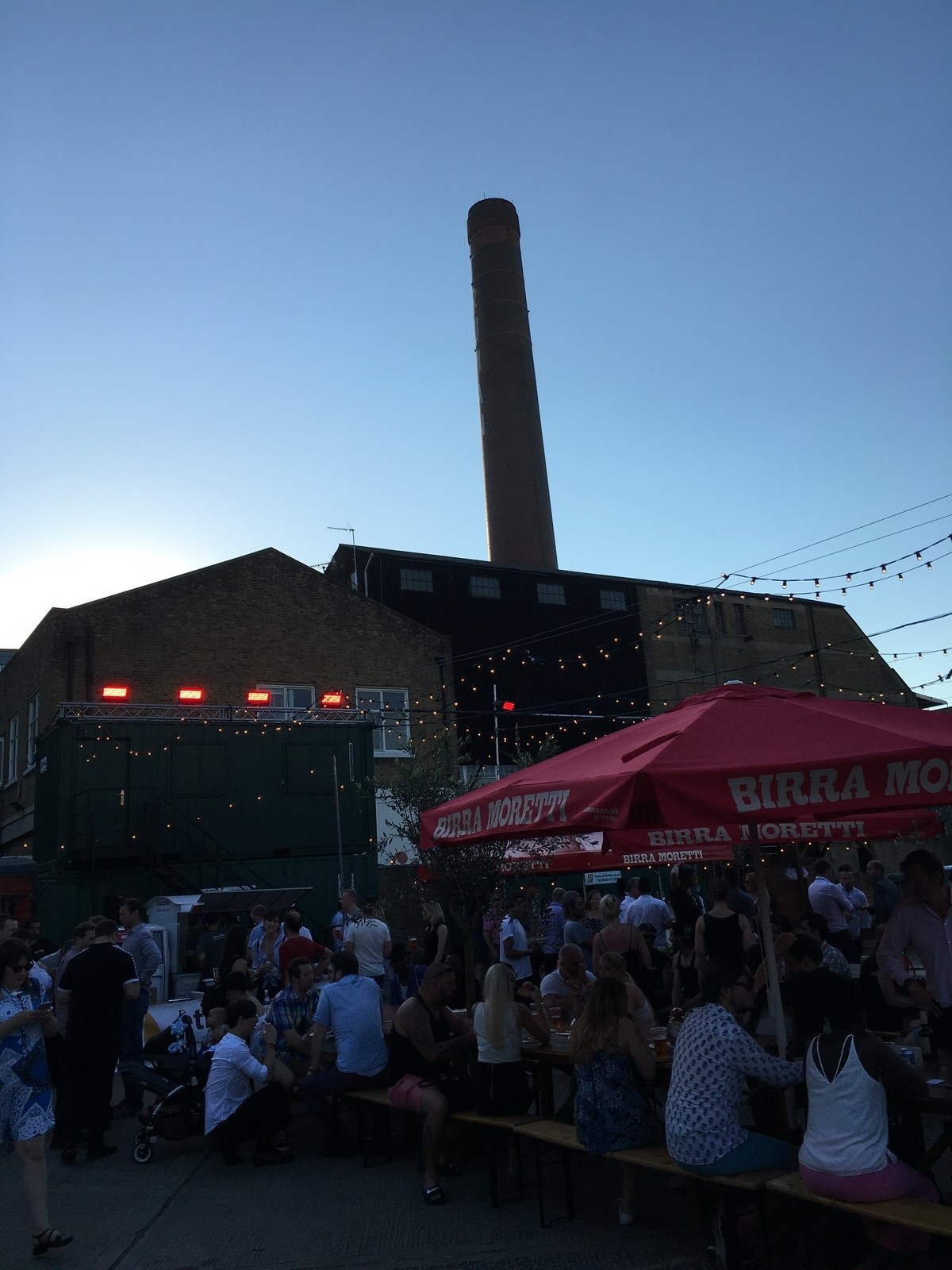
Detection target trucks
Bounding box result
[116,881,314,1054]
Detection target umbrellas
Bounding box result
[418,686,952,1129]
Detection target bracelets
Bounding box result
[309,1066,321,1074]
[267,1042,276,1048]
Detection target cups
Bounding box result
[530,1003,544,1020]
[650,1027,667,1059]
[549,1007,561,1029]
[335,930,341,938]
[885,1042,895,1052]
[409,937,417,952]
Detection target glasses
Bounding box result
[642,933,657,941]
[742,880,750,884]
[511,971,517,978]
[426,967,454,984]
[326,969,335,974]
[8,960,35,974]
[733,977,756,992]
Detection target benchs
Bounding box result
[342,1091,952,1269]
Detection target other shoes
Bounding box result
[252,1147,295,1166]
[60,1144,78,1162]
[614,1198,635,1225]
[112,1099,142,1118]
[85,1144,117,1160]
[706,1232,731,1269]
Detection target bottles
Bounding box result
[919,1010,941,1070]
[668,1007,686,1047]
[323,972,328,984]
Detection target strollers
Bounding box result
[116,1009,256,1164]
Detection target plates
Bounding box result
[522,1040,539,1043]
[555,1032,571,1035]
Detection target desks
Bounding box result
[773,1048,951,1182]
[379,1002,793,1157]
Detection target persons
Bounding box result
[0,939,77,1256]
[471,963,551,1176]
[178,913,227,995]
[571,978,664,1224]
[473,850,952,1047]
[0,893,163,1165]
[798,988,941,1253]
[199,888,477,1205]
[679,954,805,1259]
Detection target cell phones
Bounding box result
[37,1002,51,1011]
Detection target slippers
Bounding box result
[422,1184,445,1205]
[436,1159,463,1177]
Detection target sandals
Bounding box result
[32,1229,74,1255]
